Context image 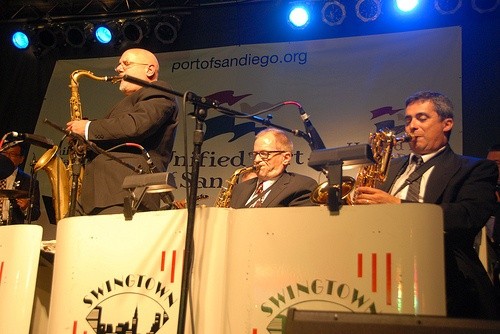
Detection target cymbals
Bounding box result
[0,152,17,181]
[0,188,29,200]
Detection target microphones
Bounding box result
[298,107,326,150]
[12,131,55,149]
[142,149,175,203]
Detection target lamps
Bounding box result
[307,144,376,212]
[11,0,499,51]
[121,172,177,220]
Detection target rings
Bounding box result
[366,200,368,205]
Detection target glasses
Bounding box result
[115,61,149,70]
[2,151,21,157]
[248,151,292,158]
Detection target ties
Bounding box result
[254,183,264,208]
[405,155,423,202]
[0,179,6,224]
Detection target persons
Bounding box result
[65,48,178,217]
[353,92,500,319]
[0,138,42,226]
[226,129,318,208]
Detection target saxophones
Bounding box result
[310,127,415,208]
[33,68,120,228]
[215,164,261,207]
